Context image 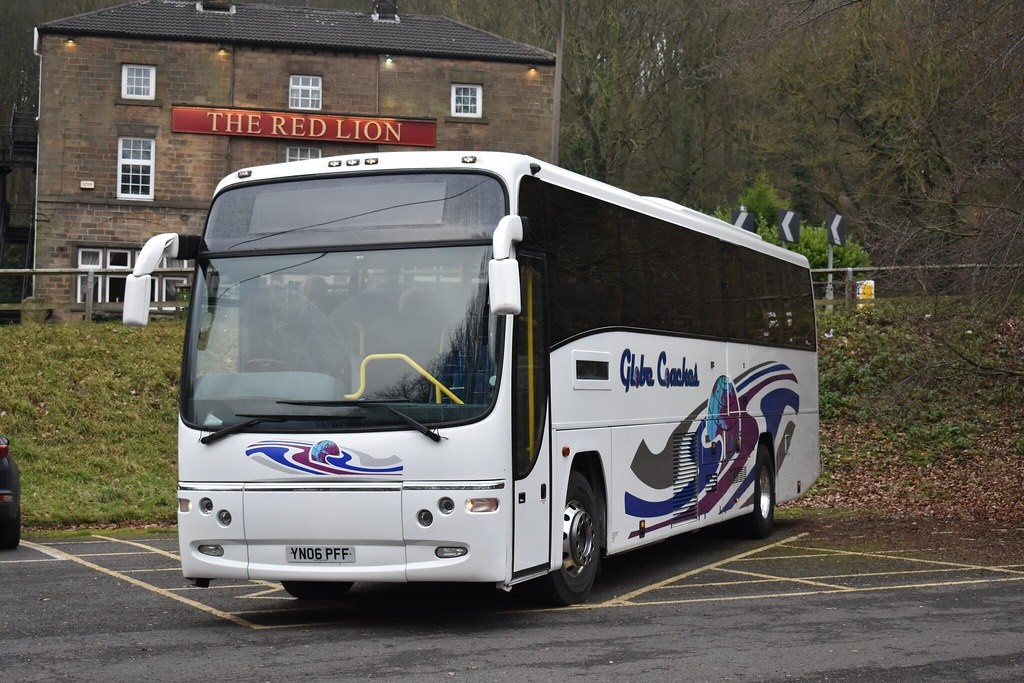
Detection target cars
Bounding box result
[0,432,22,552]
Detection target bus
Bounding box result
[120,149,820,606]
